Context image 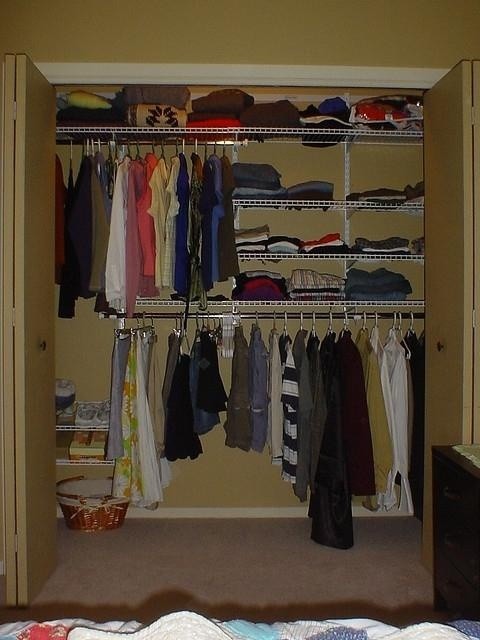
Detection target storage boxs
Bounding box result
[69,430,109,459]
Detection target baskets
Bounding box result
[56,476,129,531]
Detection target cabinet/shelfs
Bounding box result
[432,441,479,624]
[54,402,114,467]
[57,95,426,307]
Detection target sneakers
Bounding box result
[54,378,111,427]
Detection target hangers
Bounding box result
[58,127,230,173]
[120,309,416,358]
